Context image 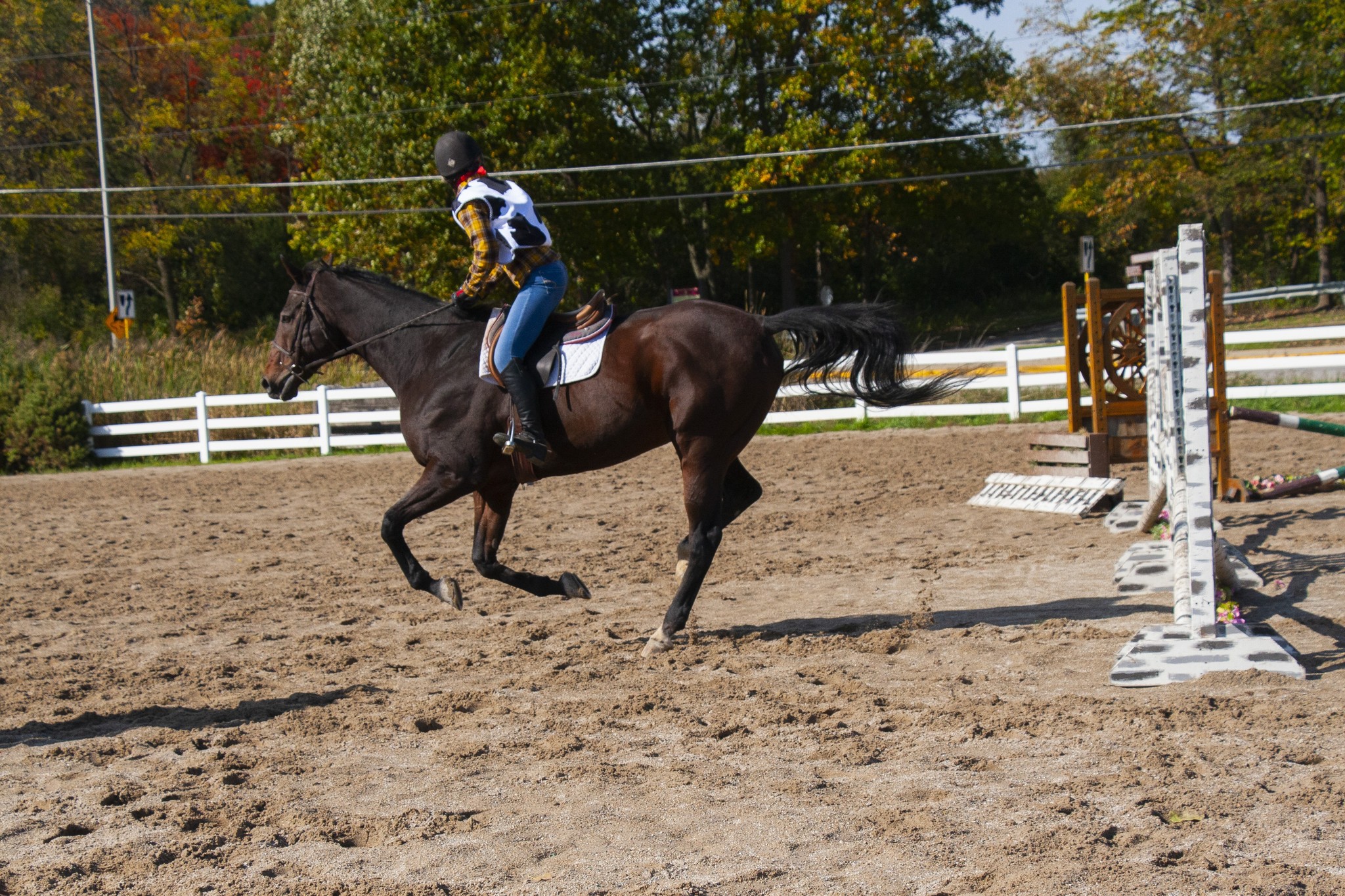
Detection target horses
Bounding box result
[259,248,989,653]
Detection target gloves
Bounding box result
[451,290,476,310]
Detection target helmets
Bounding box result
[433,129,481,178]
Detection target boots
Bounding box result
[492,357,550,468]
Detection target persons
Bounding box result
[434,132,567,466]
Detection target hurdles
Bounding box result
[1060,219,1306,688]
